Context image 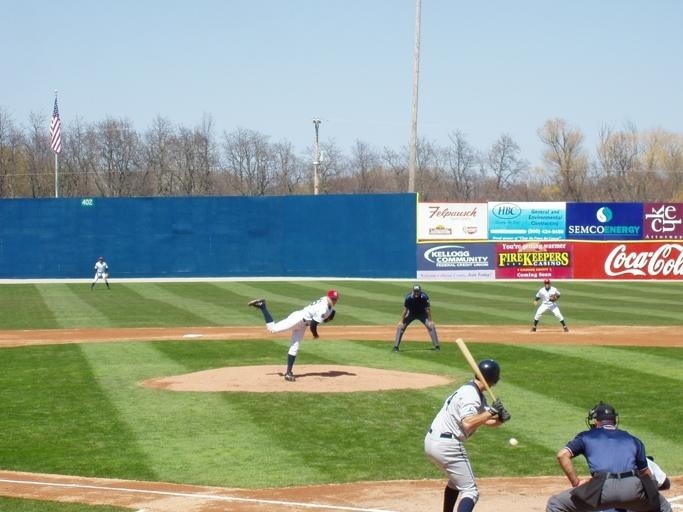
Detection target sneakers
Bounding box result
[284,373,295,382]
[530,325,568,332]
[392,346,440,352]
[247,298,266,308]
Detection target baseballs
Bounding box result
[509,438,518,447]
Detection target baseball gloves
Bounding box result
[324,310,335,323]
[550,294,560,302]
[104,268,108,273]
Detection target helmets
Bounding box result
[474,359,499,385]
[588,402,617,425]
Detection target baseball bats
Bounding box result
[456,338,497,402]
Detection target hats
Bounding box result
[412,285,420,293]
[327,290,338,300]
[543,278,551,284]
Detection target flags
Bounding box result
[48,97,62,155]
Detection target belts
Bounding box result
[302,318,308,326]
[427,428,455,438]
[607,470,633,479]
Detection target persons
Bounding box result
[90,256,110,290]
[545,397,675,511]
[422,357,511,511]
[392,283,441,352]
[245,289,341,384]
[645,455,670,490]
[530,280,570,333]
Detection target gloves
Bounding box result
[489,399,504,416]
[497,408,511,423]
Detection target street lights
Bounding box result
[312,118,322,195]
